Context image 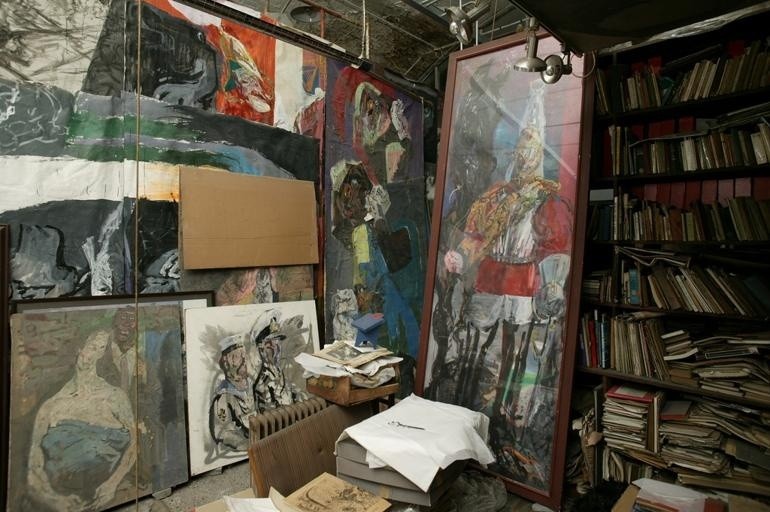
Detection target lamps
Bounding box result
[445,0,572,84]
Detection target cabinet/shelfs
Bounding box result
[575,11,770,502]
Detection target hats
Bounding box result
[251,309,286,344]
[219,332,246,355]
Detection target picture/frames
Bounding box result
[9,291,212,406]
[414,26,594,510]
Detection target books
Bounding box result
[568,32,769,511]
